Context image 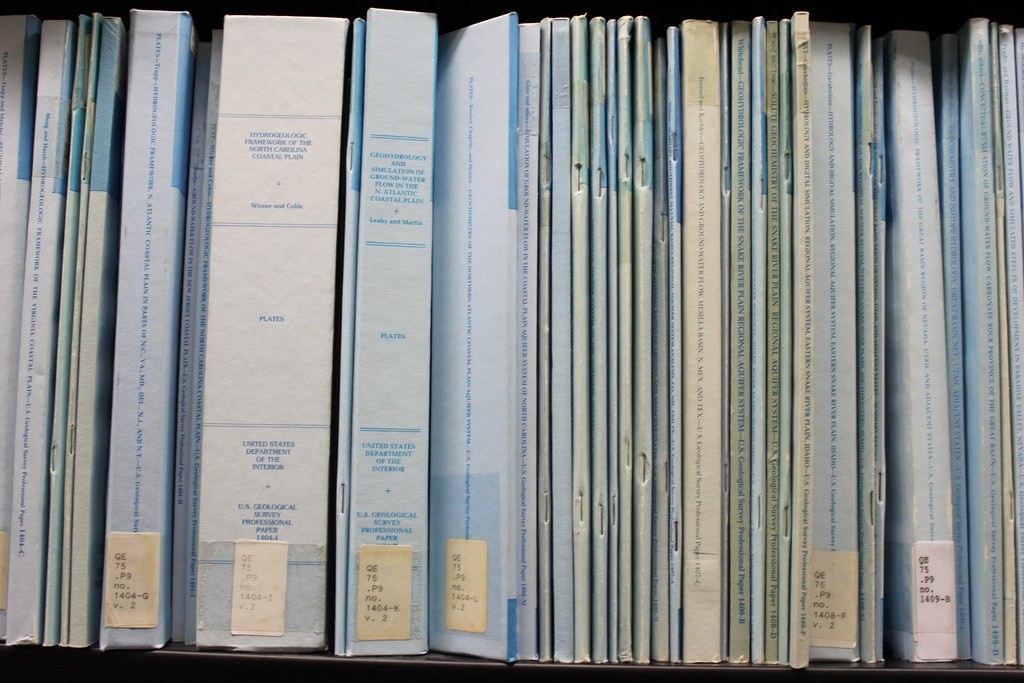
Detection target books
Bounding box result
[0,9,1024,669]
